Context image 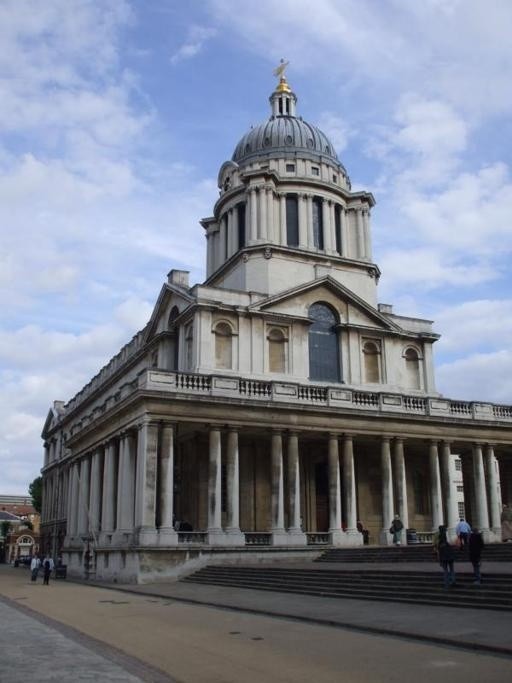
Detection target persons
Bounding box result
[30,554,42,582]
[456,518,473,550]
[436,532,458,588]
[466,526,486,585]
[42,553,55,584]
[431,525,445,560]
[391,513,403,546]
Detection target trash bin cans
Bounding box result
[56,565,66,579]
[406,528,417,544]
[15,560,19,568]
